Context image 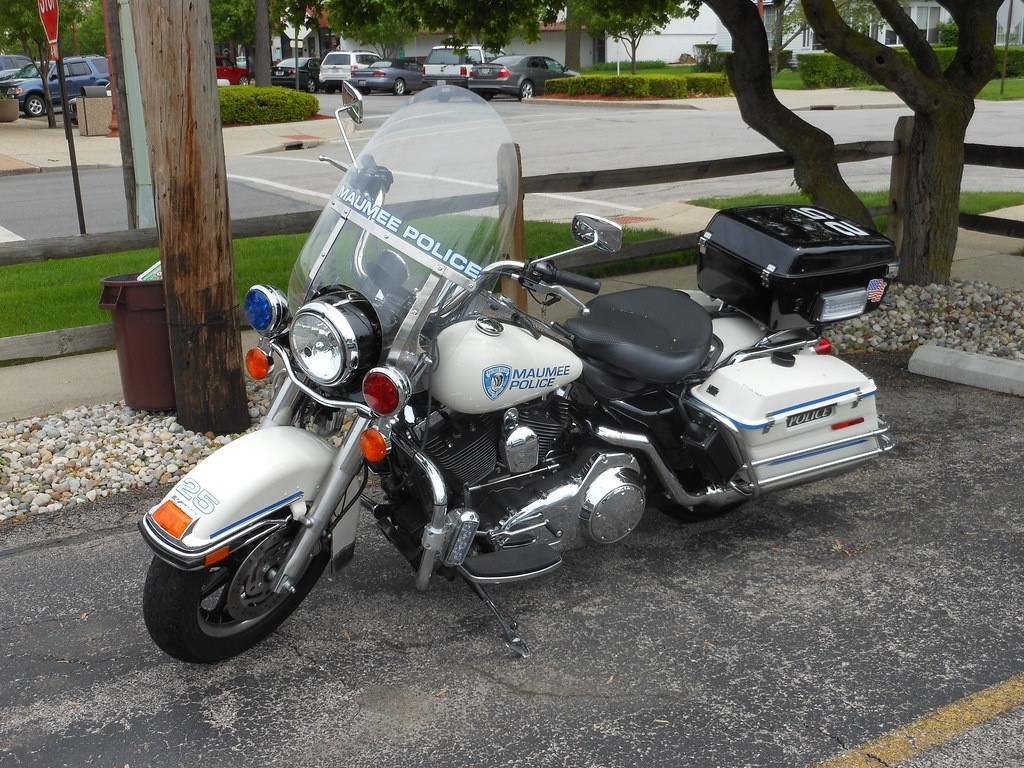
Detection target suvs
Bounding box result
[421,44,507,103]
[0,53,111,119]
[318,49,386,97]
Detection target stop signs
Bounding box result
[38,0,61,44]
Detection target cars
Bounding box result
[467,54,583,102]
[348,57,424,97]
[0,54,33,82]
[269,56,325,94]
[216,55,255,86]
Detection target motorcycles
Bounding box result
[134,79,904,667]
